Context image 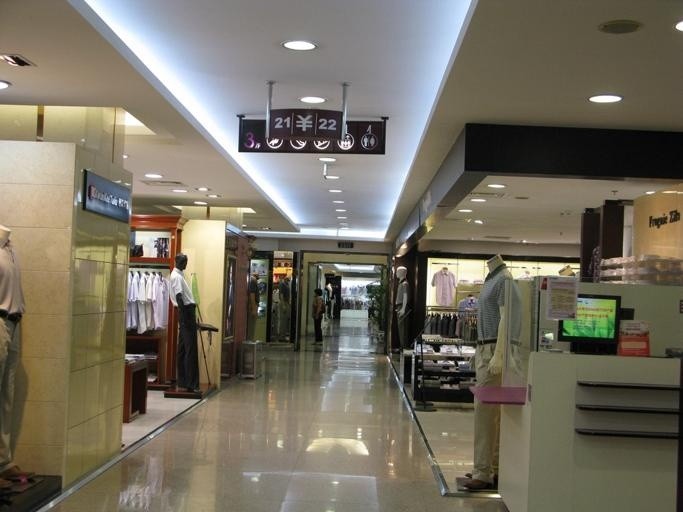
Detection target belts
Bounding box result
[475,337,498,346]
[0,308,23,324]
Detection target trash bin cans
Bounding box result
[240,340,263,380]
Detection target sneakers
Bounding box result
[0,465,36,490]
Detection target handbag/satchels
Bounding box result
[313,298,325,314]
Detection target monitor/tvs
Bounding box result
[571,307,636,355]
[559,295,621,343]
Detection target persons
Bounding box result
[462,252,523,491]
[167,252,203,395]
[0,222,39,491]
[310,287,326,345]
[324,288,328,320]
[393,265,410,355]
[275,276,290,344]
[246,271,261,342]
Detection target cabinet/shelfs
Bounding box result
[411,338,478,403]
[576,380,681,440]
[127,213,188,393]
[599,258,680,287]
